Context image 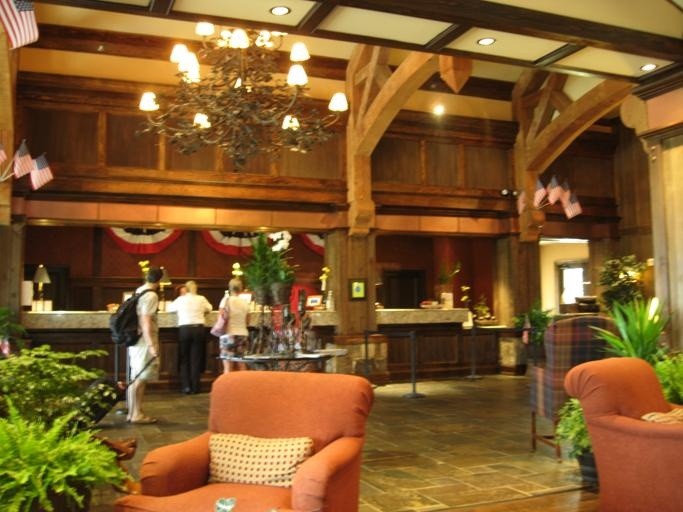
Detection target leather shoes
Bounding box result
[179,388,201,395]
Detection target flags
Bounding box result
[532,179,548,208]
[13,140,33,178]
[0,0,39,50]
[563,193,582,220]
[30,156,52,190]
[546,176,560,206]
[516,190,527,216]
[560,180,570,209]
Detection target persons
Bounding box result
[210,277,250,374]
[166,280,213,392]
[170,284,188,302]
[110,265,162,423]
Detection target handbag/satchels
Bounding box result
[209,297,230,338]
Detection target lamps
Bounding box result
[135,21,349,172]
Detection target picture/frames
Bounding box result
[348,278,366,301]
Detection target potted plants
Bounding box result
[242,231,301,308]
[0,343,117,511]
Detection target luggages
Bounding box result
[75,355,158,432]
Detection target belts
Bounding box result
[179,324,203,328]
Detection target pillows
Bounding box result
[207,432,313,490]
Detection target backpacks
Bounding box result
[108,288,159,349]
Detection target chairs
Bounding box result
[112,370,373,512]
[526,315,682,512]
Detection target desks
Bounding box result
[218,344,347,373]
